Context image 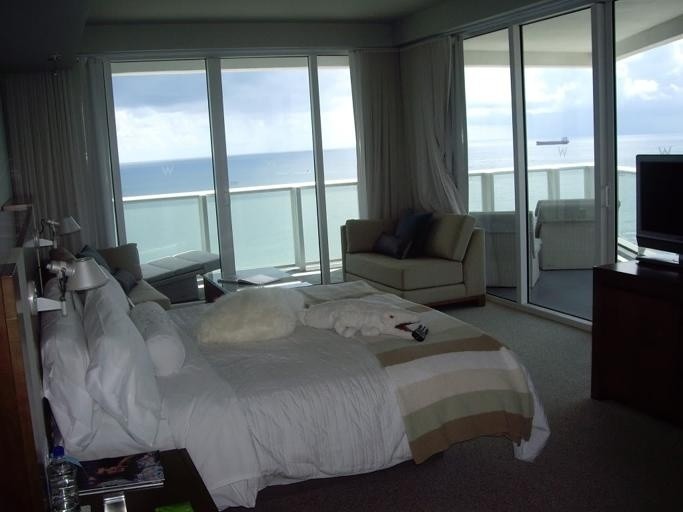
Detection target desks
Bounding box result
[201,265,313,303]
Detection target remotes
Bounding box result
[412,323,429,342]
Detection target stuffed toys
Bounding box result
[294,298,420,341]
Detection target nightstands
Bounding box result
[53,448,219,511]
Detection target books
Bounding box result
[77,450,166,497]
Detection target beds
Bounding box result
[0,204,550,511]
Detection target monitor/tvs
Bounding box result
[635,154,683,270]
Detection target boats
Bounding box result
[536,136,570,145]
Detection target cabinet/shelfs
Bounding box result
[591,259,683,428]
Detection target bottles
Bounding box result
[45,445,82,512]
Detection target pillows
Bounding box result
[345,206,475,261]
[194,287,303,346]
[38,243,185,450]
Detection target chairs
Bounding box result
[535,199,620,269]
[341,212,486,306]
[469,211,542,288]
[125,249,222,310]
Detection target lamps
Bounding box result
[28,257,110,317]
[35,215,82,250]
